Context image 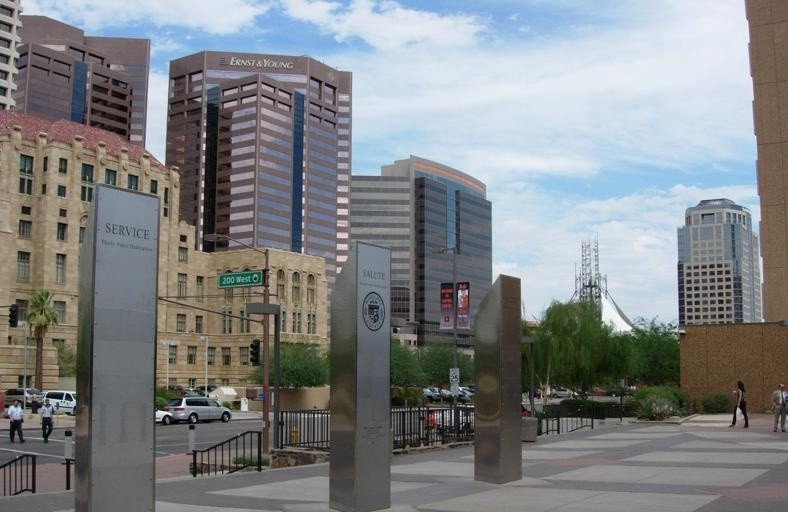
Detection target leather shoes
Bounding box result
[774,428,785,432]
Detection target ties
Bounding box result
[781,392,785,406]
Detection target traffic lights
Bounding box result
[10,304,18,327]
[250,339,260,363]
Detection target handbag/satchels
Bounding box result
[736,407,744,420]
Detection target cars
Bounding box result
[548,386,578,399]
[155,409,174,425]
[433,409,475,434]
[424,386,474,403]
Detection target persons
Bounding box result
[39,399,54,443]
[773,384,787,433]
[8,400,26,444]
[424,410,435,441]
[729,380,748,428]
[520,406,528,416]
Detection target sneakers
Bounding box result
[728,424,734,427]
[43,435,48,443]
[11,438,24,442]
[744,425,748,428]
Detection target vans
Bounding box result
[7,388,43,408]
[164,397,232,423]
[44,391,77,415]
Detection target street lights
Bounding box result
[200,336,207,396]
[204,234,270,454]
[163,342,170,391]
[433,247,458,366]
